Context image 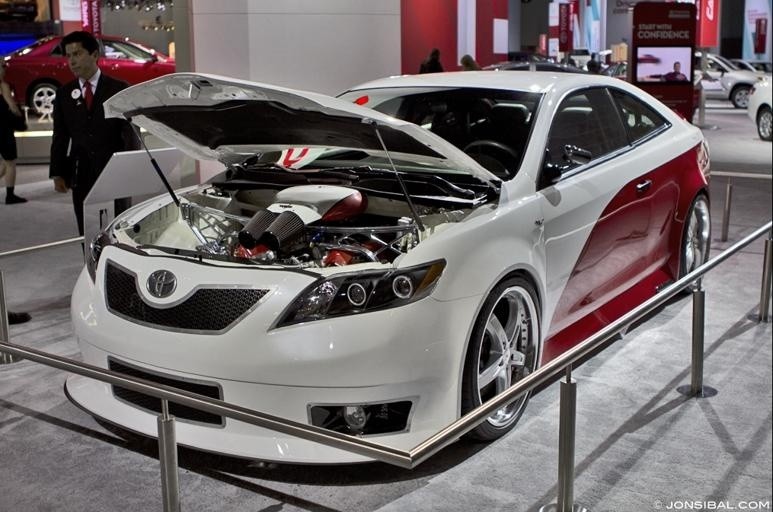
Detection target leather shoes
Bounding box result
[7,310,31,325]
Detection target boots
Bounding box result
[5,187,27,204]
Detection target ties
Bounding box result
[83,80,94,110]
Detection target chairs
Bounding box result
[475,100,592,149]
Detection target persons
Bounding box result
[614,37,629,65]
[586,53,602,74]
[662,63,687,80]
[0,80,29,203]
[49,29,142,258]
[460,55,483,70]
[560,51,576,67]
[420,49,443,71]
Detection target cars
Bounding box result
[602,61,699,113]
[482,51,586,74]
[695,51,773,141]
[64,70,711,471]
[3,34,175,118]
